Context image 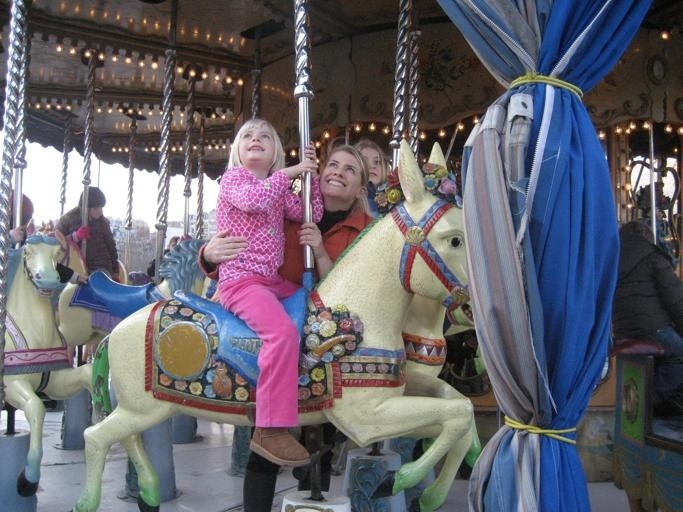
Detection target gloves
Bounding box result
[76,225,90,241]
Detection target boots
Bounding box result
[249,425,310,466]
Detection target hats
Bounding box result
[79,187,105,208]
[11,191,33,225]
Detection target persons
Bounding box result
[195,144,374,512]
[608,219,682,417]
[7,188,35,251]
[352,140,395,220]
[216,118,325,467]
[55,185,120,283]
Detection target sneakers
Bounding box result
[652,395,683,417]
